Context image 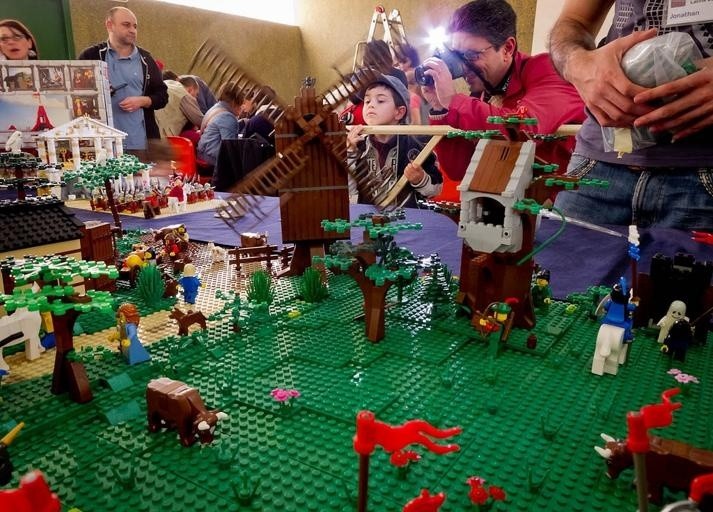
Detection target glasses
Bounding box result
[0,34,26,42]
[450,44,496,61]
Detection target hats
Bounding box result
[351,69,409,110]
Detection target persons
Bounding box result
[76,5,169,167]
[145,1,710,337]
[109,302,153,366]
[0,17,39,60]
[126,223,207,305]
[592,279,697,366]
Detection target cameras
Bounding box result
[415,49,467,86]
